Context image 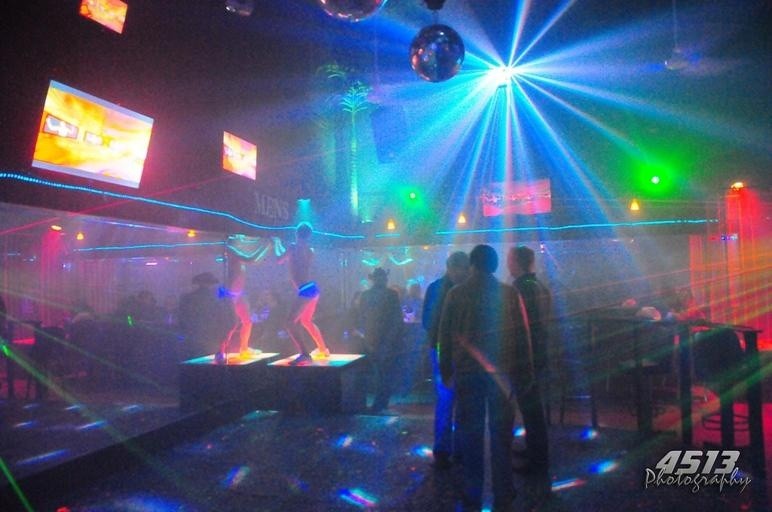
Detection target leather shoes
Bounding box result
[510,446,551,476]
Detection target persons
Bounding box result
[420,250,475,468]
[502,246,556,476]
[274,222,329,366]
[216,233,273,363]
[346,264,409,414]
[437,243,536,507]
[666,286,707,320]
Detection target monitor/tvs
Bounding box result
[478,175,554,219]
[219,127,260,184]
[26,72,160,195]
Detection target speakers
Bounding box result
[370,105,419,163]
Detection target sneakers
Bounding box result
[288,353,313,364]
[311,347,331,359]
[239,347,262,358]
[214,352,226,364]
